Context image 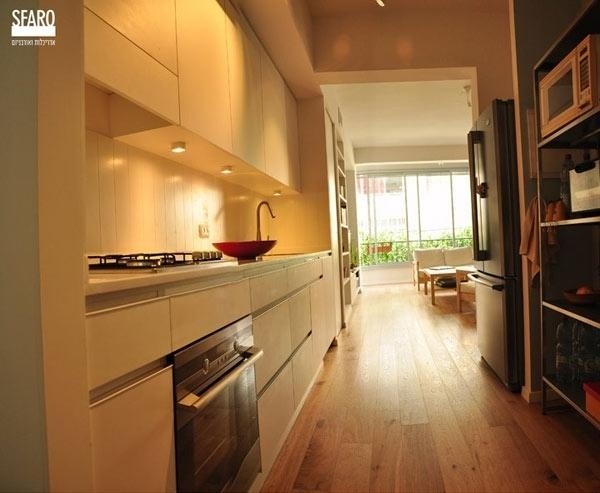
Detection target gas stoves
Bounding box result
[88,251,223,269]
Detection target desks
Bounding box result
[423,266,476,305]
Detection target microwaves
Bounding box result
[536,40,600,136]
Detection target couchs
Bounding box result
[412,247,477,291]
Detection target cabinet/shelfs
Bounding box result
[86,256,342,493]
[334,124,354,329]
[81,0,302,197]
[531,1,600,436]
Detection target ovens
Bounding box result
[173,315,263,493]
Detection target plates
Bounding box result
[558,285,596,303]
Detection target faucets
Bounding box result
[255,199,275,262]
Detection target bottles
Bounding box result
[556,151,594,217]
[555,320,591,382]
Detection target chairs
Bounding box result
[455,269,476,312]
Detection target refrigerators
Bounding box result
[466,98,523,394]
